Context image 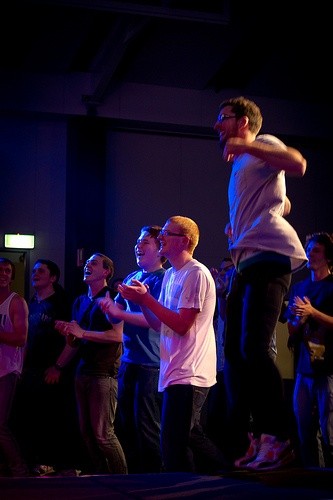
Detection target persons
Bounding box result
[117,216,218,473]
[0,258,29,478]
[99,226,168,474]
[211,257,304,465]
[55,254,127,474]
[9,258,71,476]
[287,232,333,483]
[214,99,310,471]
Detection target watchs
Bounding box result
[55,363,65,371]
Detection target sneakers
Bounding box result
[234,432,262,470]
[246,434,295,470]
[30,463,56,477]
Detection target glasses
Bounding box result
[160,229,186,236]
[219,114,237,121]
